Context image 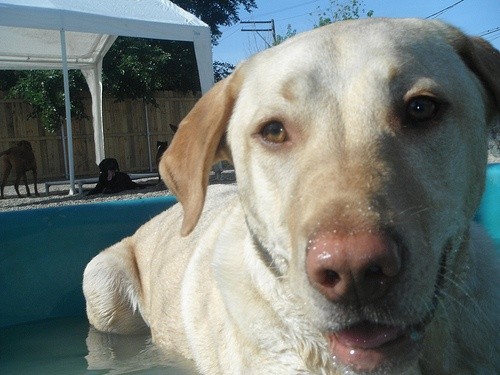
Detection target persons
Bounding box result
[86,158,135,196]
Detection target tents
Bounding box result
[1,0,224,198]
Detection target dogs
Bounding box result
[80,16,500,374]
